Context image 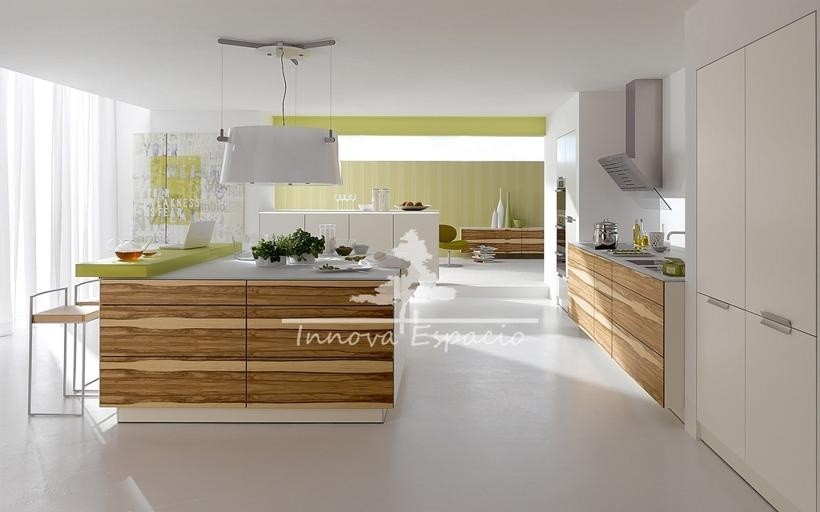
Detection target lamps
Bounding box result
[439,224,468,267]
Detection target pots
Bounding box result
[592,218,621,250]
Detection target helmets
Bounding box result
[394,204,431,211]
[313,264,374,273]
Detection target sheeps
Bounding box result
[667,229,685,241]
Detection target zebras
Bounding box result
[555,176,566,277]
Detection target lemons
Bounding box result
[105,237,153,261]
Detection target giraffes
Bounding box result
[604,246,655,256]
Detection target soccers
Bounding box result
[402,201,422,206]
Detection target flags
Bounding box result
[491,187,512,228]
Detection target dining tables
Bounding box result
[248,228,327,268]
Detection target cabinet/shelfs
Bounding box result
[633,218,650,246]
[371,187,390,210]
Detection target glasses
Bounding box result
[217,37,343,188]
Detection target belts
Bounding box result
[335,193,357,210]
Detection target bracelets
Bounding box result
[653,247,667,252]
[358,203,373,212]
[353,244,370,255]
[330,239,356,256]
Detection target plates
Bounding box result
[460,227,544,254]
[543,89,661,316]
[692,7,819,512]
[566,240,685,408]
[259,210,439,283]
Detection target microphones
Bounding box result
[27,279,100,417]
